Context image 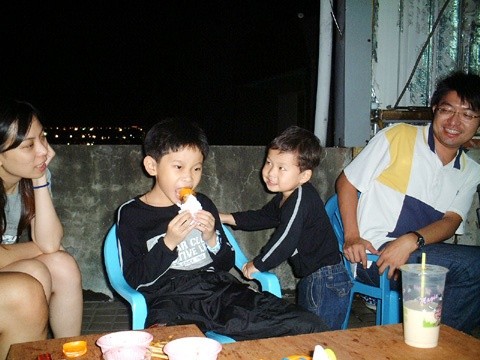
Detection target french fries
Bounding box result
[148,341,168,359]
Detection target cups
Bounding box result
[163,337,222,360]
[96,331,154,360]
[399,262,449,349]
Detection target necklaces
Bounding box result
[144,195,148,204]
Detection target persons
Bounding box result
[335,72,479,342]
[0,100,85,360]
[218,128,352,330]
[117,119,324,342]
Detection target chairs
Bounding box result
[104,224,281,329]
[325,190,399,328]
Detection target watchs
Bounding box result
[407,231,424,250]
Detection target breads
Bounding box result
[179,188,195,203]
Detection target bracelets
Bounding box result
[33,182,49,189]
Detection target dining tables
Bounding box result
[6,323,480,360]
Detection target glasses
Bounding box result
[436,107,480,122]
